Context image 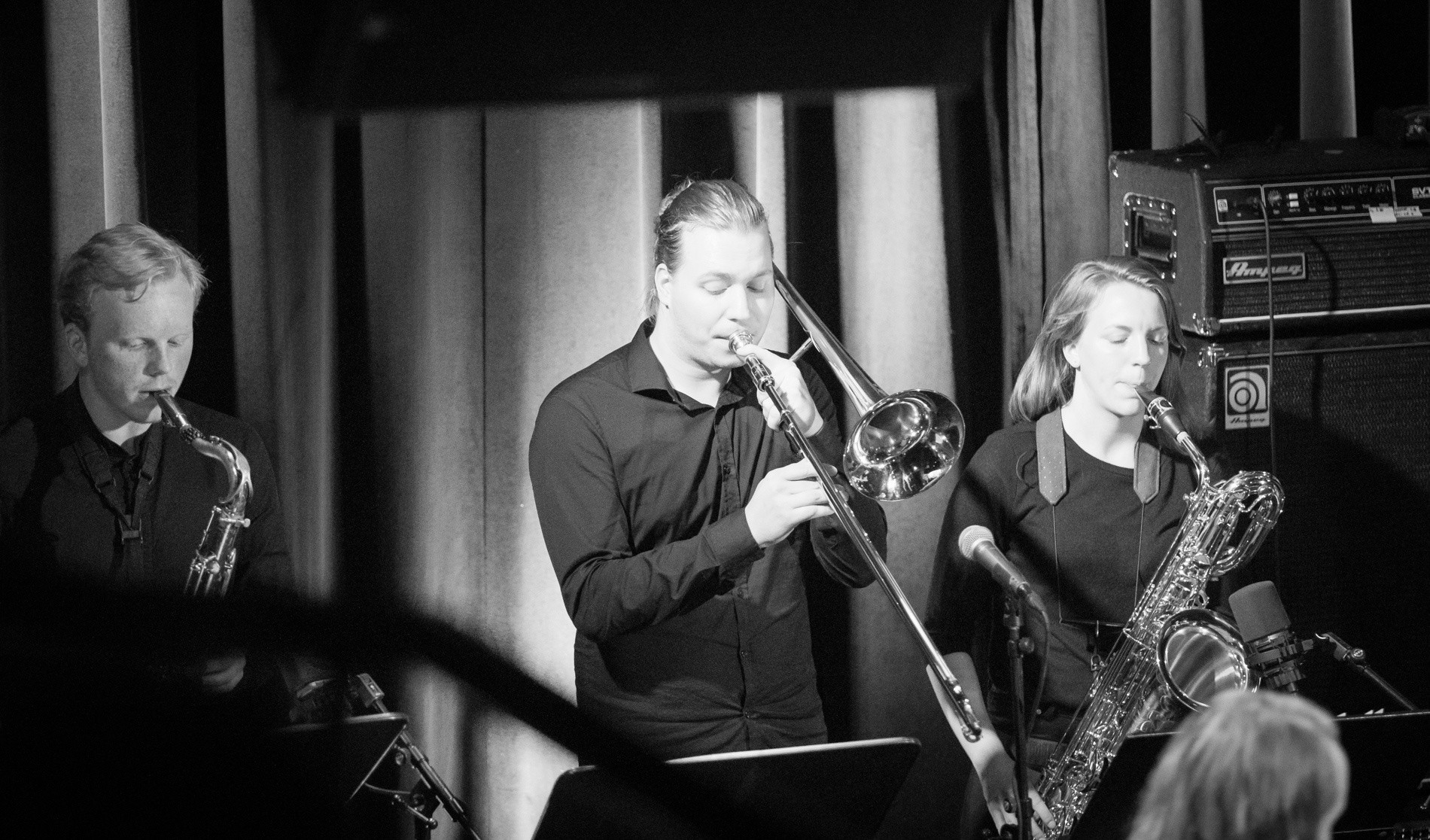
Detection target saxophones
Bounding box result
[150,389,257,601]
[1028,382,1286,840]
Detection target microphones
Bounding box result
[1228,579,1307,692]
[959,523,1048,615]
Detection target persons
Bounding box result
[0,220,311,729]
[920,253,1241,840]
[1126,685,1353,840]
[527,178,888,768]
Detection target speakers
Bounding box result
[1152,329,1429,840]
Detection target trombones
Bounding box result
[727,262,983,746]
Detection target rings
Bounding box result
[1002,799,1018,812]
